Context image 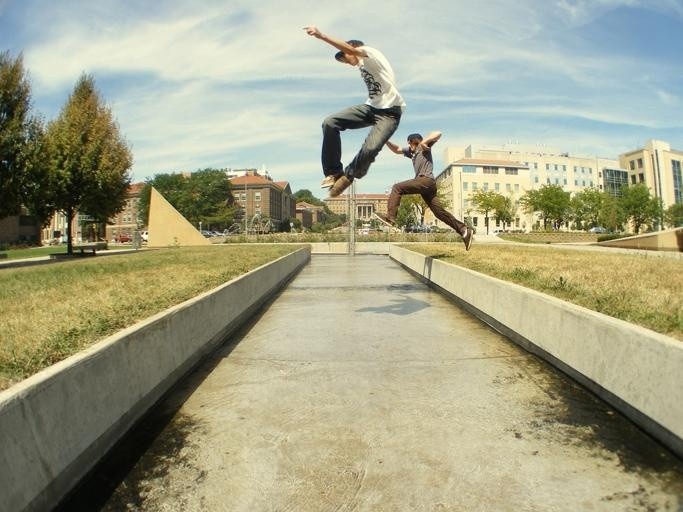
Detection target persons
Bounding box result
[303,25,406,198]
[374,131,474,250]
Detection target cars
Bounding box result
[115,231,134,243]
[588,226,606,234]
[404,223,430,233]
[201,230,224,238]
[429,226,449,233]
[448,227,457,233]
[140,230,149,243]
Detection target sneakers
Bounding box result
[320,169,344,188]
[327,175,351,197]
[460,226,474,251]
[374,212,395,227]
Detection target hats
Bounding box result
[335,40,364,63]
[407,133,423,140]
